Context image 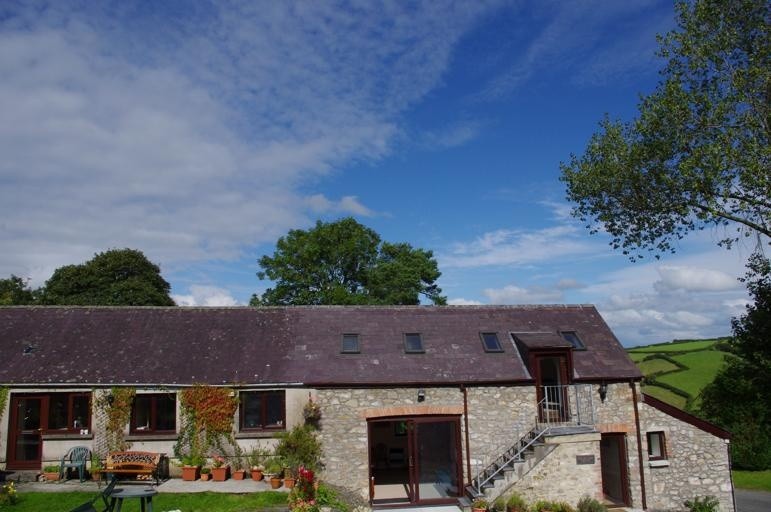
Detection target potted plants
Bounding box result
[466,495,556,512]
[36,423,331,488]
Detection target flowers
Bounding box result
[0,477,21,501]
[284,463,319,512]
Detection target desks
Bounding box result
[106,485,161,512]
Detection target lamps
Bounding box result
[418,389,426,402]
[598,376,609,404]
